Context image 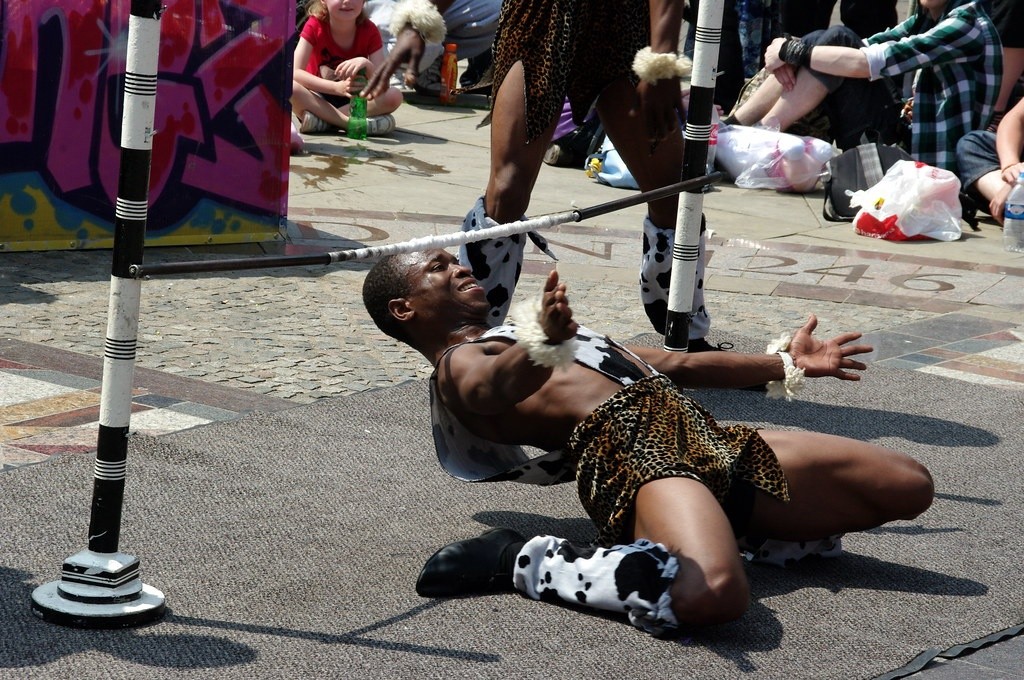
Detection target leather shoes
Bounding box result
[416,527,525,598]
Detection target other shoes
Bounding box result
[689,341,725,354]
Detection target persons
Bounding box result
[290,0,1024,352]
[363,247,935,633]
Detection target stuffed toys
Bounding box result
[716,127,832,193]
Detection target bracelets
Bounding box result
[779,33,814,69]
[511,298,579,371]
[631,46,692,85]
[1001,164,1017,173]
[766,332,805,402]
[388,0,447,46]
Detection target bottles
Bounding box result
[440,43,458,106]
[1002,172,1024,254]
[346,68,368,141]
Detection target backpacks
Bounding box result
[818,129,917,223]
[585,120,640,191]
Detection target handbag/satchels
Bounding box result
[844,158,962,243]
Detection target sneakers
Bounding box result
[299,110,328,133]
[366,114,396,135]
[415,58,446,96]
